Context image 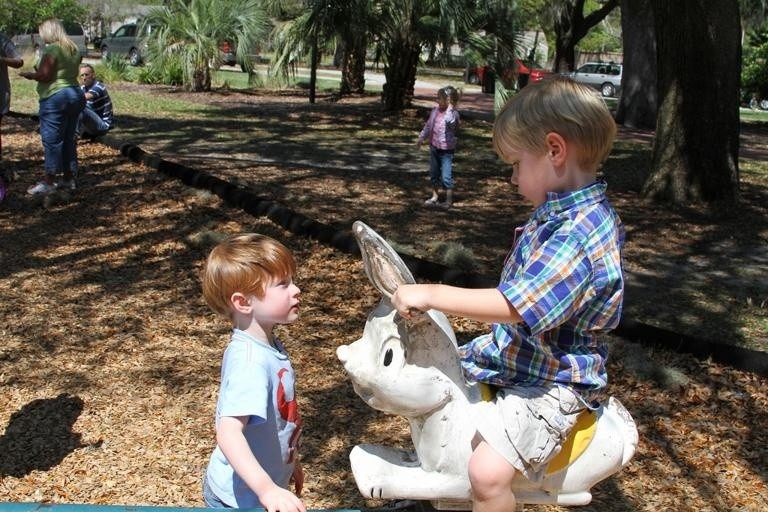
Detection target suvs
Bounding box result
[566,62,623,96]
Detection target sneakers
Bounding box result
[27,179,75,195]
[424,199,452,209]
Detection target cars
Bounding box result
[465,57,546,91]
[207,37,260,74]
[100,23,162,65]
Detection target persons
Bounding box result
[202,233,306,512]
[73,63,114,144]
[417,86,462,210]
[18,17,86,194]
[0,33,23,201]
[391,77,626,512]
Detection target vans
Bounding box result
[10,21,87,63]
